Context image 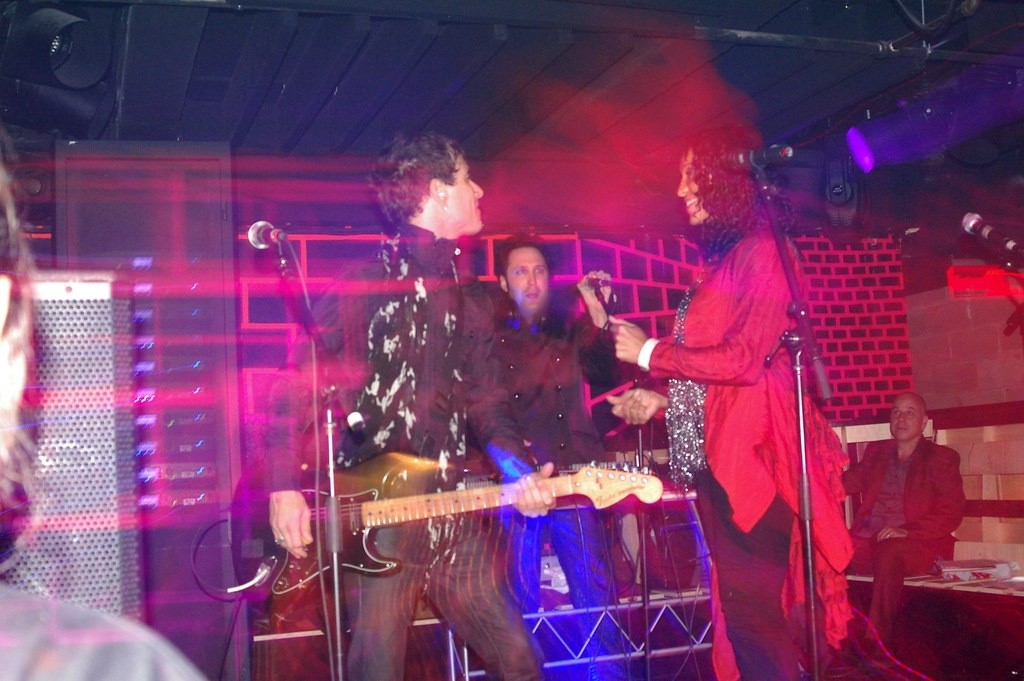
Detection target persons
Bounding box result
[608,123,852,681]
[843,392,966,668]
[273,142,617,680]
[0,163,211,678]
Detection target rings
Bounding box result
[275,536,284,544]
[886,534,890,539]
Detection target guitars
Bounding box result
[270,457,667,597]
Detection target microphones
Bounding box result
[725,143,793,173]
[248,220,288,249]
[582,275,611,289]
[962,212,1021,253]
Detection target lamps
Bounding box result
[825,159,852,202]
[0,6,113,91]
